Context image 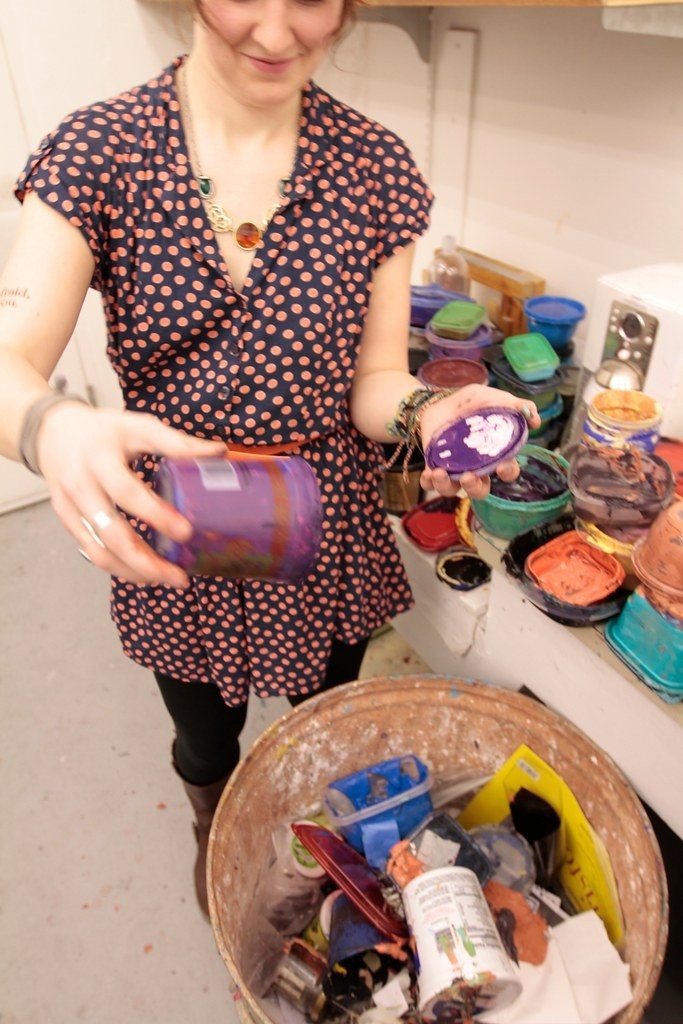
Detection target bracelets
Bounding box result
[20,396,86,474]
[380,387,453,483]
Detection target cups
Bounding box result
[401,865,523,1021]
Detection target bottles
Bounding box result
[427,237,469,297]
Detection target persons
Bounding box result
[1,0,542,919]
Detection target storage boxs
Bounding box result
[428,299,486,341]
[411,286,479,327]
[601,591,683,704]
[492,356,559,410]
[500,332,562,384]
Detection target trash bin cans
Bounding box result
[206,674,670,1024]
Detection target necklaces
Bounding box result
[183,63,300,251]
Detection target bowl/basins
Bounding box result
[151,457,323,586]
[382,282,683,703]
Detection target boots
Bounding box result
[171,737,227,923]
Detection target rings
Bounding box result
[94,512,116,534]
